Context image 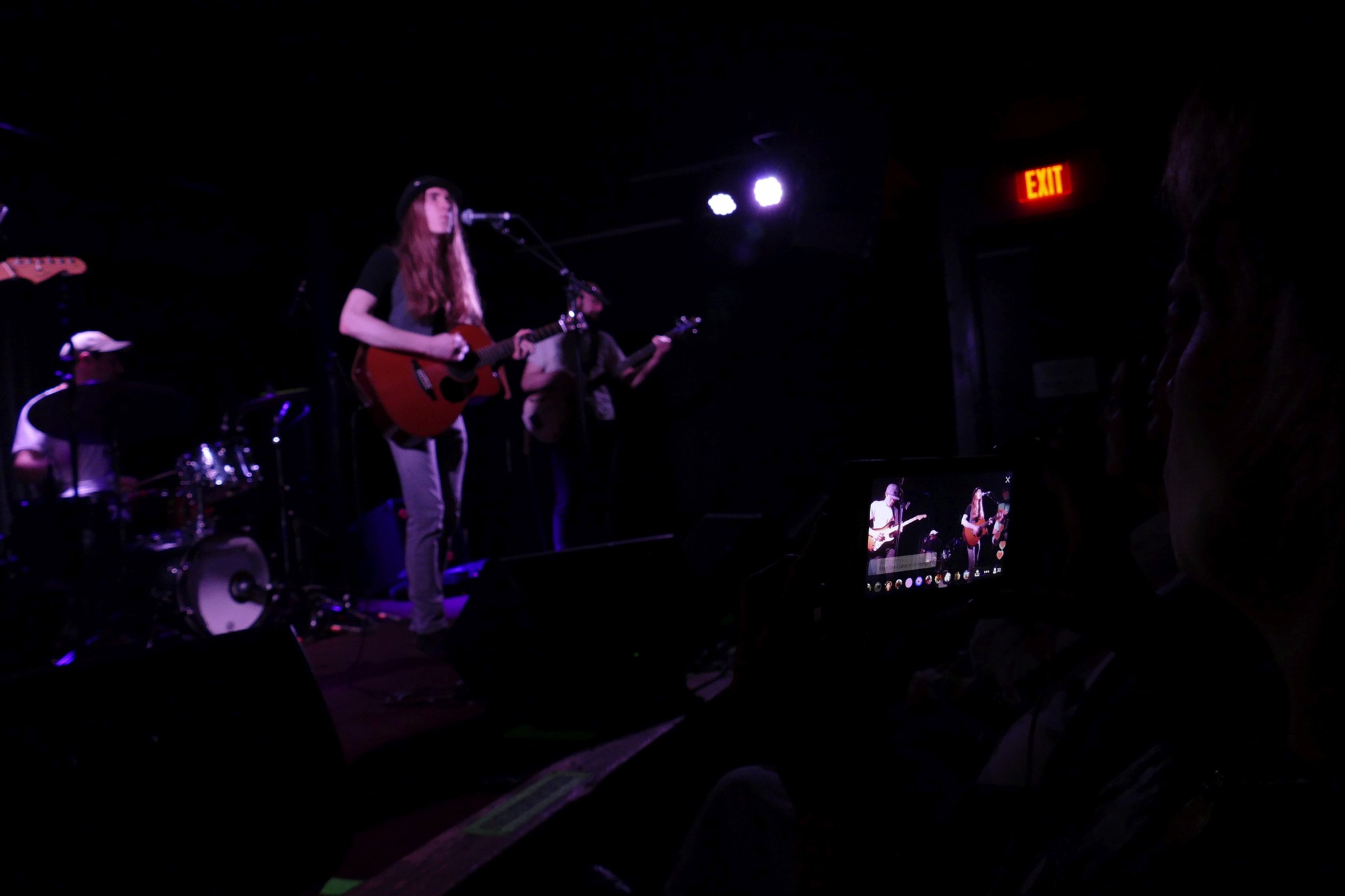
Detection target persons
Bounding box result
[959,486,1009,582]
[869,483,904,575]
[11,330,140,521]
[336,174,535,654]
[1096,120,1344,886]
[519,280,667,554]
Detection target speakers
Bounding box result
[0,533,705,896]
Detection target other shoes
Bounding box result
[409,619,449,634]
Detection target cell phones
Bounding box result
[830,457,1046,609]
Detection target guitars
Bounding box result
[357,310,589,441]
[520,313,704,446]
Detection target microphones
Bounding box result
[462,208,518,226]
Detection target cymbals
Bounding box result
[27,382,171,446]
[241,383,320,410]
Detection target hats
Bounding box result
[972,486,983,496]
[61,328,130,357]
[395,177,446,222]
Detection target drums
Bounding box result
[175,438,263,505]
[119,478,220,555]
[166,541,272,639]
